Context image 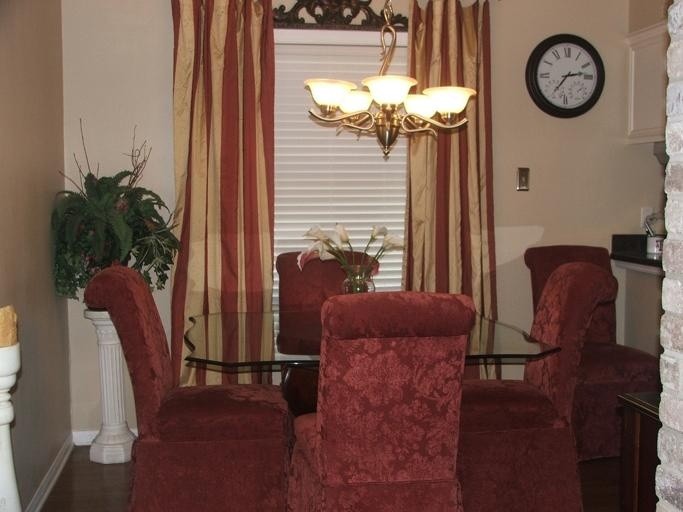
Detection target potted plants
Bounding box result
[52,122,182,465]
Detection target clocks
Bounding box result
[525,32,607,121]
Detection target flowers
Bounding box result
[296,222,404,292]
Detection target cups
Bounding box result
[646,236,664,254]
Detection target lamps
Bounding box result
[303,1,478,161]
